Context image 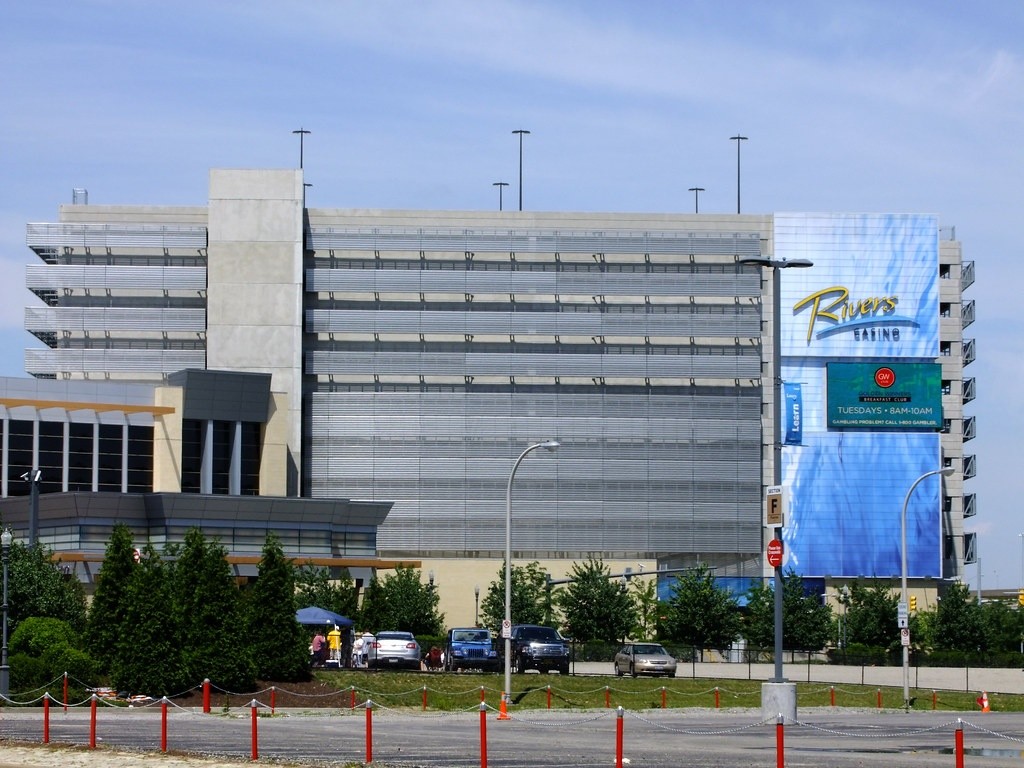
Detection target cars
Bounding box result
[614,642,678,679]
[366,631,424,670]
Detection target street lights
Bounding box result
[292,126,311,169]
[428,570,435,613]
[474,583,480,625]
[689,186,706,213]
[745,250,814,719]
[897,466,956,706]
[0,526,14,707]
[504,442,562,703]
[492,178,510,210]
[512,129,531,211]
[729,133,748,214]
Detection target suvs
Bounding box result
[495,624,572,675]
[444,627,501,673]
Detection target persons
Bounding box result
[327,626,342,660]
[314,631,325,668]
[423,646,444,670]
[351,627,374,668]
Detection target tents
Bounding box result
[296,606,356,668]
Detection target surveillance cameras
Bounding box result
[20,472,29,478]
[34,471,42,482]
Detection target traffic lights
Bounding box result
[909,594,917,611]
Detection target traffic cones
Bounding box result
[496,691,511,721]
[980,691,992,712]
